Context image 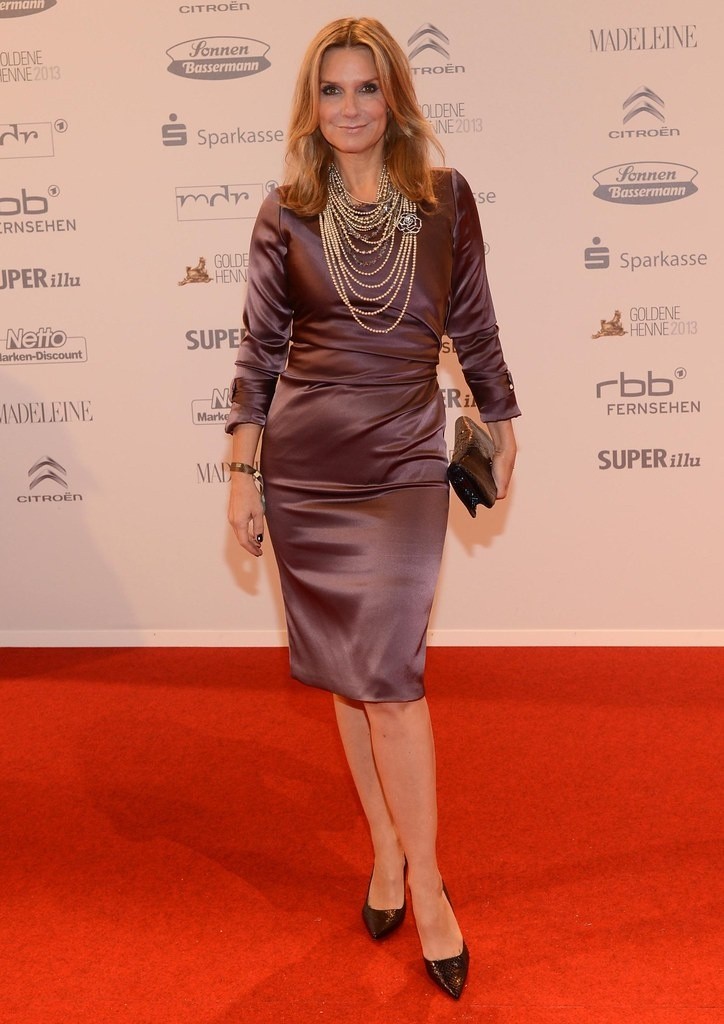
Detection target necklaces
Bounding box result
[318,159,422,334]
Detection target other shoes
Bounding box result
[412,879,469,1000]
[362,853,407,940]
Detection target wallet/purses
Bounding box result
[447,416,498,519]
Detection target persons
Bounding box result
[224,14,522,999]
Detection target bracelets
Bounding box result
[230,461,265,494]
